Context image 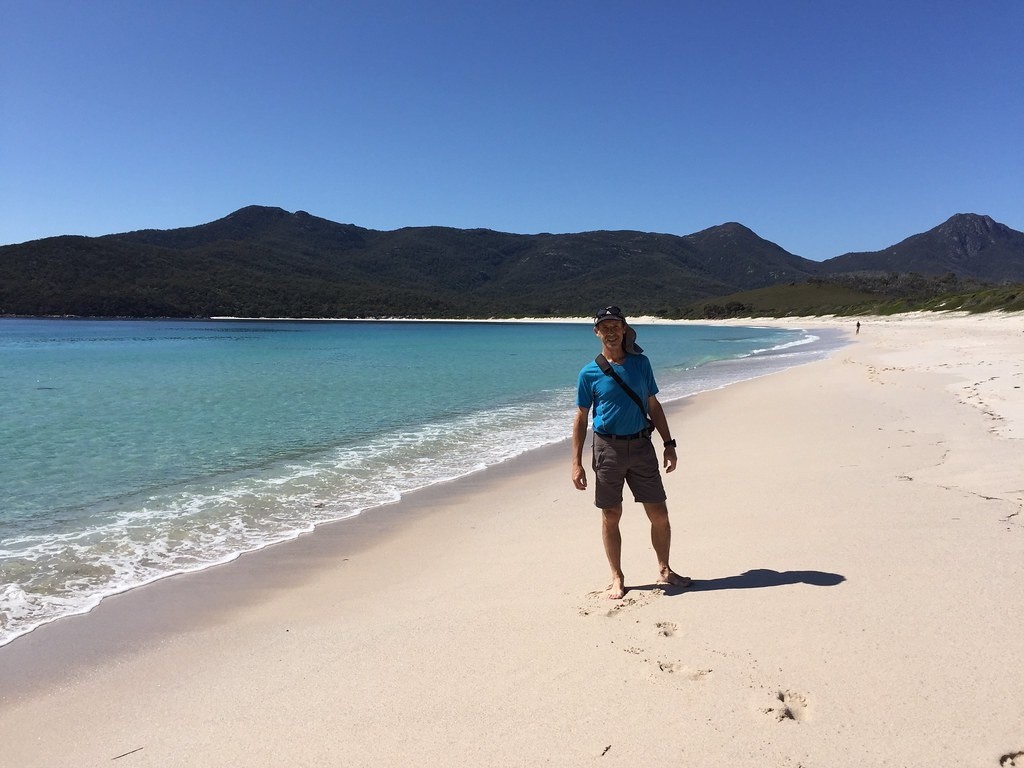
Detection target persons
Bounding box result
[857,321,860,329]
[573,305,691,599]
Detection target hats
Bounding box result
[594,305,624,325]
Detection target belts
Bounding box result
[594,428,646,440]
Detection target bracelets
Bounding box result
[664,439,676,447]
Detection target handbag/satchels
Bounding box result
[646,418,655,435]
